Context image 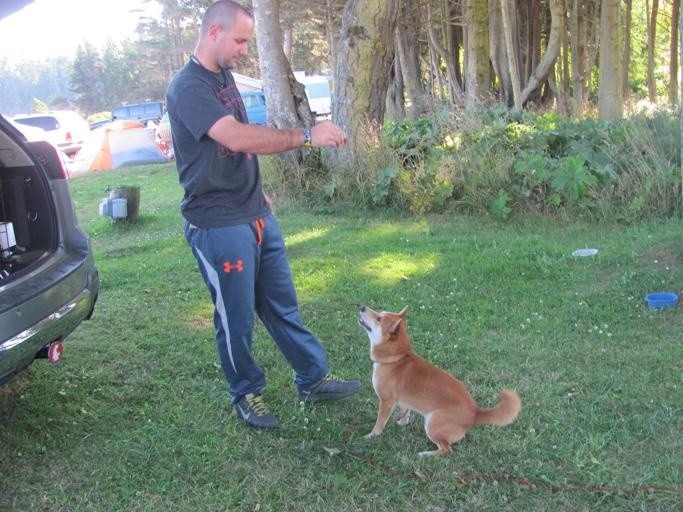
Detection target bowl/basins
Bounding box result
[645,291,679,311]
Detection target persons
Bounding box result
[163,1,365,430]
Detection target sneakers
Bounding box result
[234,392,279,428]
[296,374,362,403]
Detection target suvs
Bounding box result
[91,100,164,132]
[10,108,93,153]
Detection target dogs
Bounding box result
[357,305,522,459]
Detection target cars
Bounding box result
[238,89,269,129]
[0,0,104,407]
[298,76,332,121]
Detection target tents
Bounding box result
[70,120,165,172]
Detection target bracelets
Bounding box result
[302,128,312,152]
